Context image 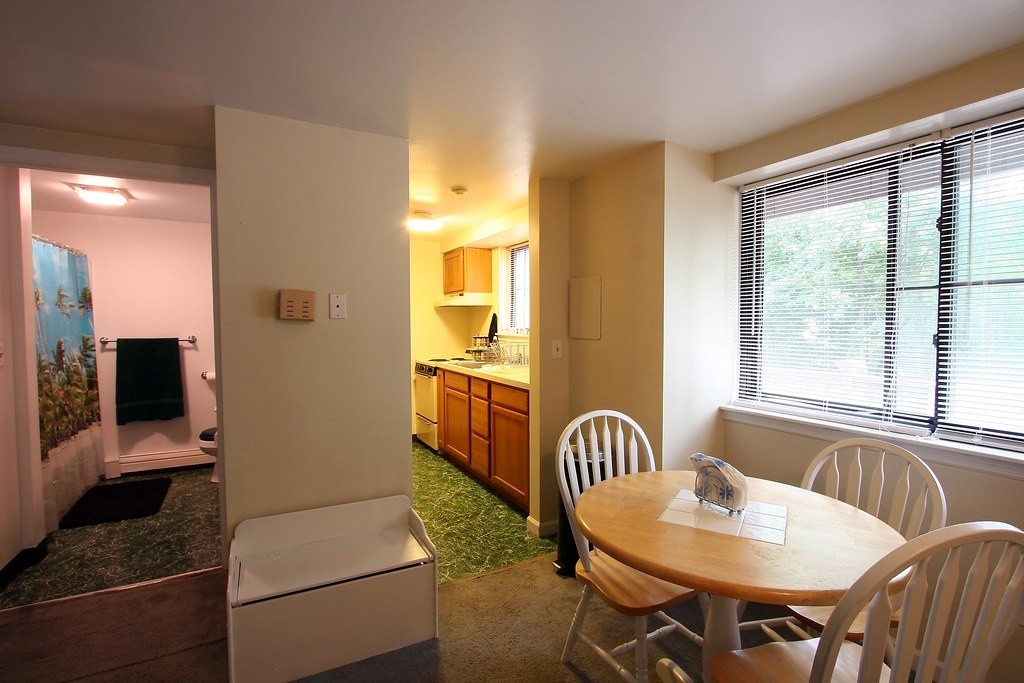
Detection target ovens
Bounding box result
[415,361,439,451]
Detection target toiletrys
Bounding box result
[115,338,185,425]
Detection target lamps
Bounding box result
[409,212,441,229]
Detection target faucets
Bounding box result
[465,349,484,354]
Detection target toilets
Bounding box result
[199,427,218,484]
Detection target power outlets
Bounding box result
[552,340,562,358]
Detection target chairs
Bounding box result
[737,437,947,669]
[553,407,710,683]
[704,520,1024,683]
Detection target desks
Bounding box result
[574,470,913,683]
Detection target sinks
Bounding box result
[448,362,486,369]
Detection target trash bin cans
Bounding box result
[551,441,614,576]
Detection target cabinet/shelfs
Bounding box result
[436,369,530,514]
[443,248,493,295]
[226,493,440,683]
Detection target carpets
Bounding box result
[57,477,171,530]
